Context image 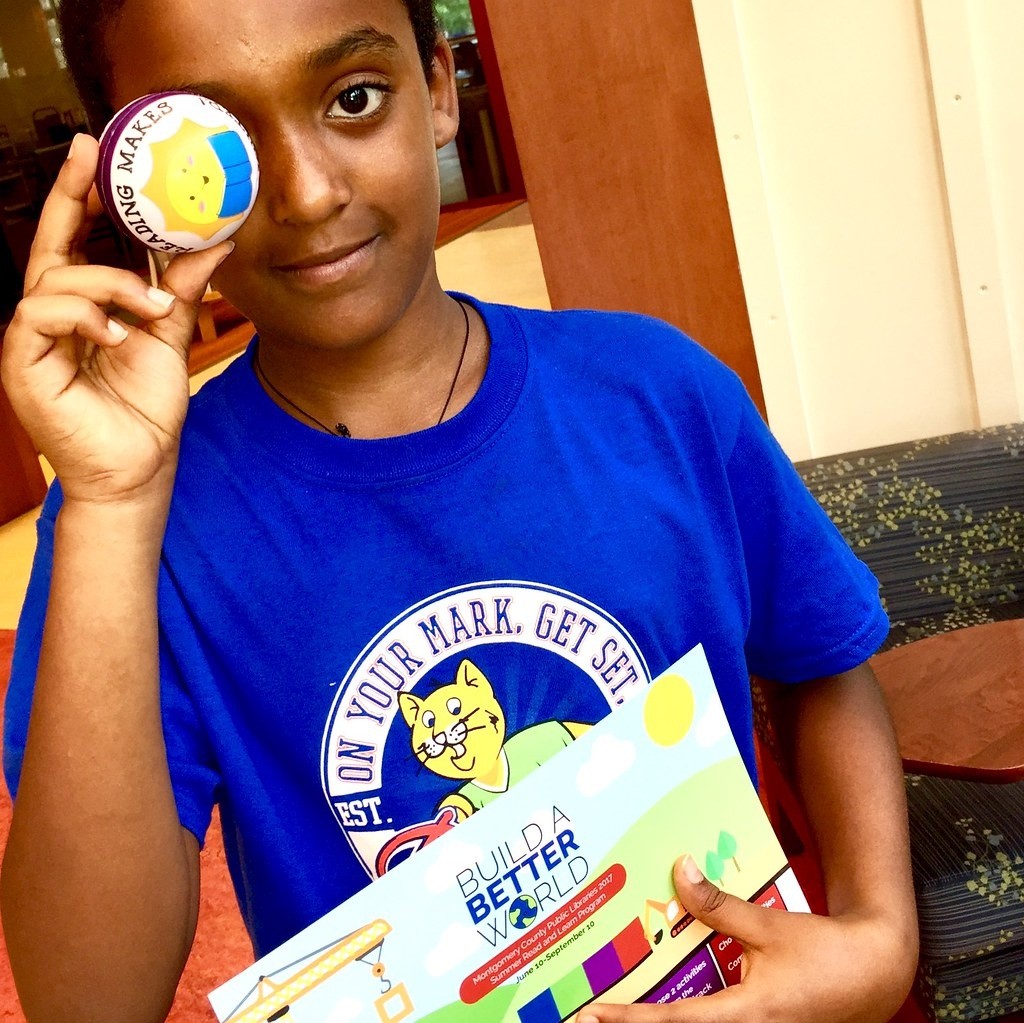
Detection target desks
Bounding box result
[861,618,1023,781]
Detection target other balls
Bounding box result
[94,89,259,256]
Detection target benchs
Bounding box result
[767,423,1024,1023]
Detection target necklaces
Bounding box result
[255,295,469,440]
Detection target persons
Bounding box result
[0,0,918,1023]
